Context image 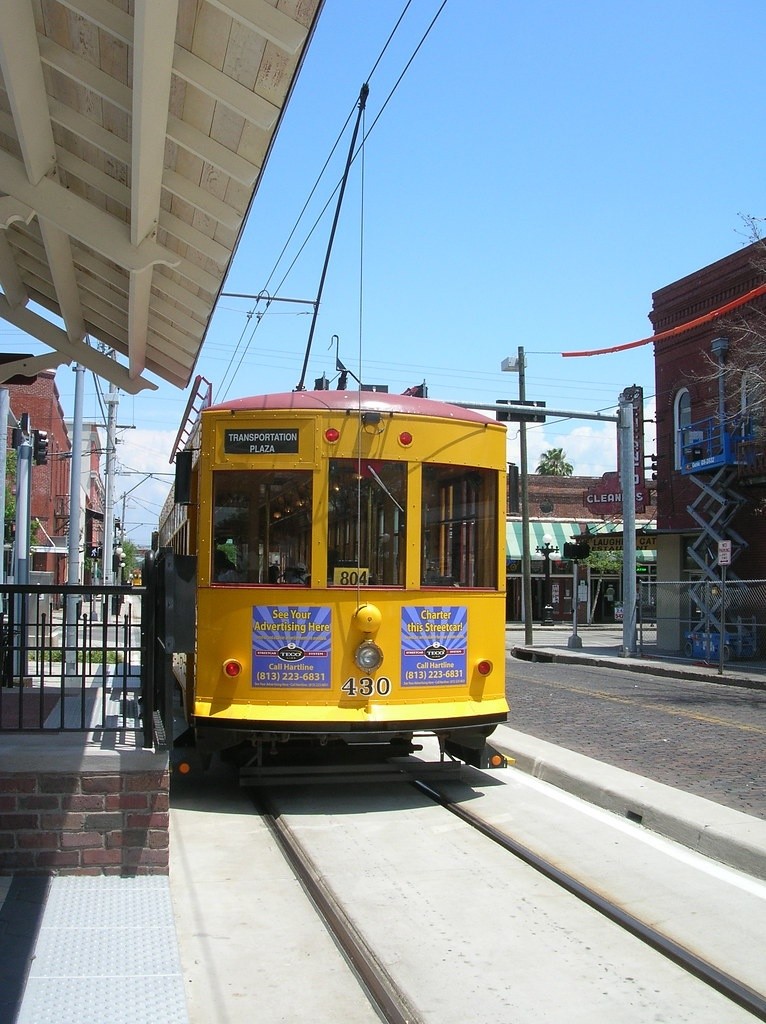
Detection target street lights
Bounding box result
[499,346,533,645]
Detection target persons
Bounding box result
[327,548,343,577]
[215,549,309,584]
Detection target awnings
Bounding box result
[505,521,657,562]
[0,1,322,391]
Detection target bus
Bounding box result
[129,568,142,586]
[145,328,521,788]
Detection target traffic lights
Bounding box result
[85,545,102,559]
[33,428,47,461]
[36,437,49,467]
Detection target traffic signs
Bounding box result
[717,539,732,567]
